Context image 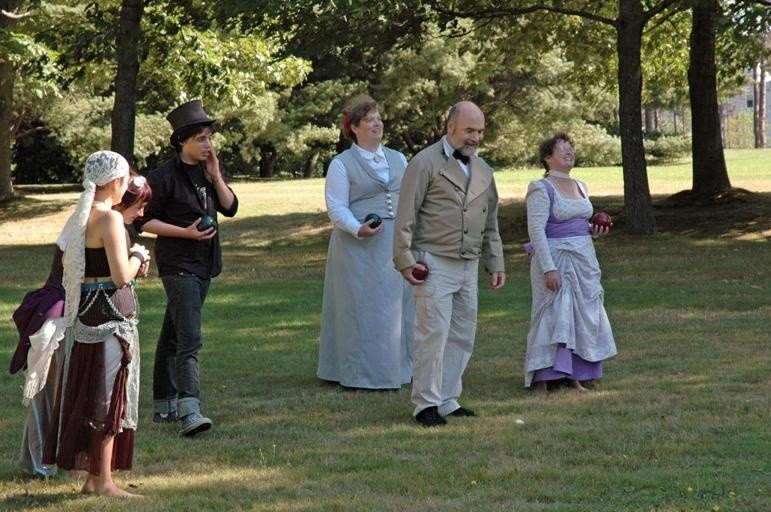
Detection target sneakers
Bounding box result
[414,405,449,432]
[178,412,215,434]
[153,412,179,424]
[448,405,482,421]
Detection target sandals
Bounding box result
[564,382,592,395]
[532,383,556,400]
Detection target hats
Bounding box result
[164,100,218,148]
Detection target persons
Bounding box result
[9,169,153,479]
[392,101,508,428]
[133,99,239,439]
[21,149,153,499]
[314,99,416,392]
[522,132,618,393]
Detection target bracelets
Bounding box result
[128,251,144,263]
[213,174,222,186]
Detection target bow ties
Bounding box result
[452,148,472,169]
